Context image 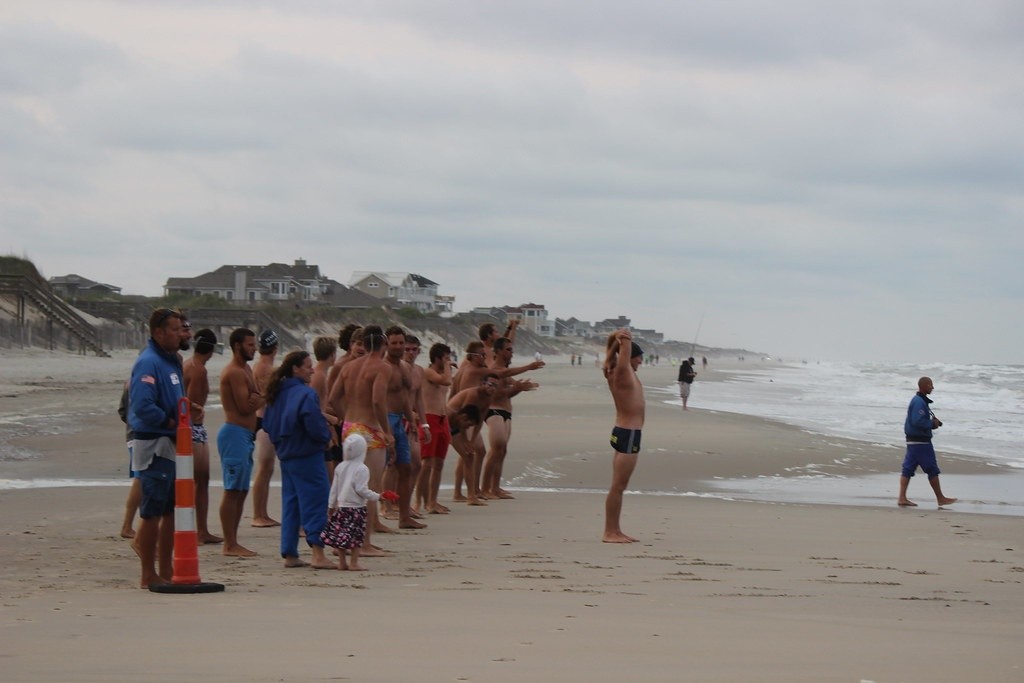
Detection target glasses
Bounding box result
[181,321,193,330]
[480,353,487,359]
[501,347,514,352]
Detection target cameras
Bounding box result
[932,417,942,429]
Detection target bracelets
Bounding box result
[422,424,429,428]
[505,326,511,330]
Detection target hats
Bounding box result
[689,357,695,365]
[259,330,277,348]
[631,342,644,359]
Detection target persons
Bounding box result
[263,316,545,568]
[602,331,644,543]
[571,352,582,366]
[644,352,659,366]
[703,357,706,368]
[182,329,224,545]
[678,357,697,408]
[898,377,958,506]
[320,434,386,571]
[252,330,281,527]
[116,308,204,589]
[219,327,266,556]
